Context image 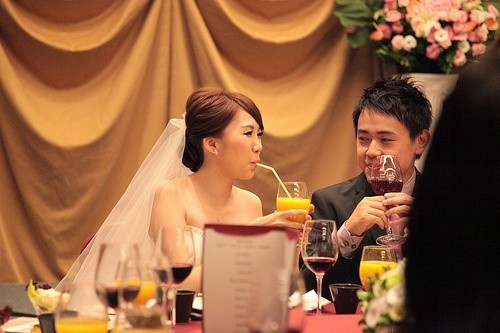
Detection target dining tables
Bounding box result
[174,303,390,333]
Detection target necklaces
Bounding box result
[214,214,228,225]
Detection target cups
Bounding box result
[115,261,154,307]
[276,182,310,211]
[176,289,195,323]
[329,284,361,314]
[359,246,397,290]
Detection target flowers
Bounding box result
[356,261,404,333]
[333,0,500,70]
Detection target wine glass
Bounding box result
[301,220,338,315]
[96,243,141,333]
[371,155,406,246]
[154,226,195,320]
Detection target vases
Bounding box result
[406,74,459,173]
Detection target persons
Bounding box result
[52,86,315,313]
[298,74,433,333]
[402,22,500,333]
